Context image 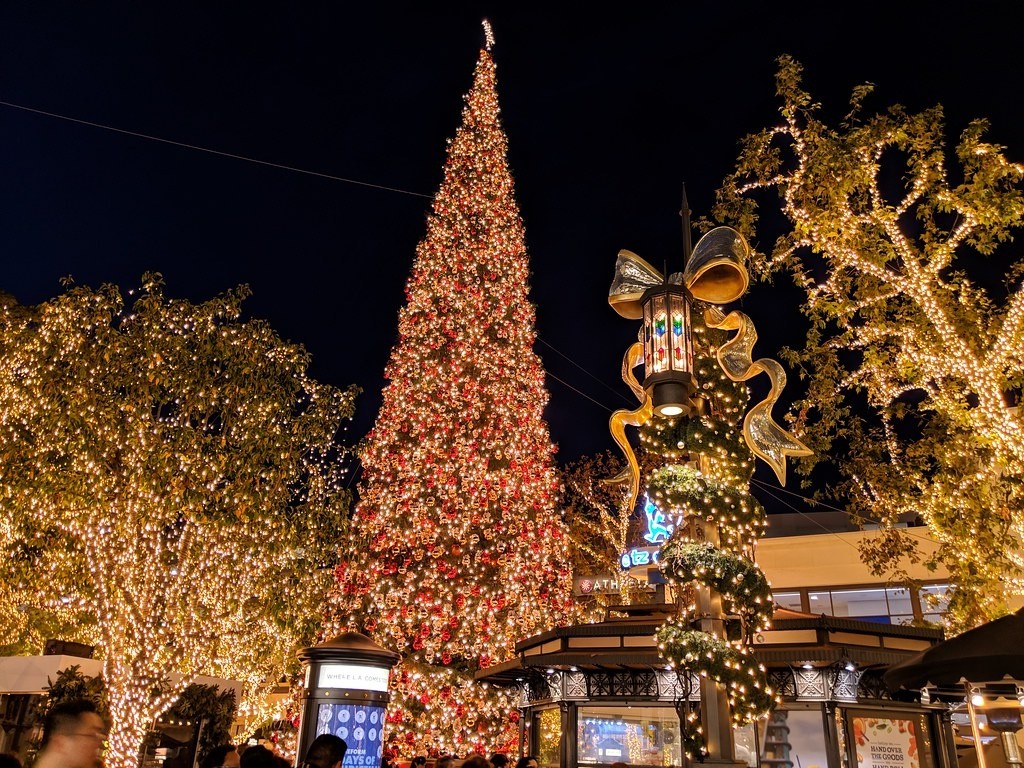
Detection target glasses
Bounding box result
[384,757,392,761]
[62,732,105,743]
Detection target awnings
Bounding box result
[884,606,1024,768]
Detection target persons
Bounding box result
[0,699,347,768]
[380,753,538,768]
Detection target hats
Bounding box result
[384,754,394,759]
